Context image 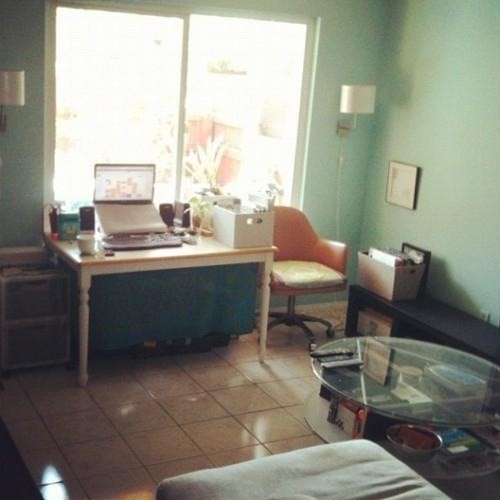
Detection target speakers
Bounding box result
[80,207,94,230]
[160,204,173,226]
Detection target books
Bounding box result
[423,364,487,456]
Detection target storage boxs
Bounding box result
[213,204,273,249]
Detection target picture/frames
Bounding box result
[363,336,395,386]
[385,161,420,210]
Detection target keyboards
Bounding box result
[99,233,182,249]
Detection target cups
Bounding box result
[76,234,96,254]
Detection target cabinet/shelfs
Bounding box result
[0,248,74,372]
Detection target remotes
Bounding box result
[310,347,353,359]
[321,358,364,369]
[104,249,113,255]
[318,354,350,362]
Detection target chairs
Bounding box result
[267,206,349,341]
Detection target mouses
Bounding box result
[181,236,196,245]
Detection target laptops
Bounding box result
[94,164,167,235]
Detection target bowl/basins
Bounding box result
[385,423,444,464]
[400,365,423,384]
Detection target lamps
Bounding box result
[1,70,26,135]
[336,85,377,136]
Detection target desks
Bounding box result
[44,231,281,387]
